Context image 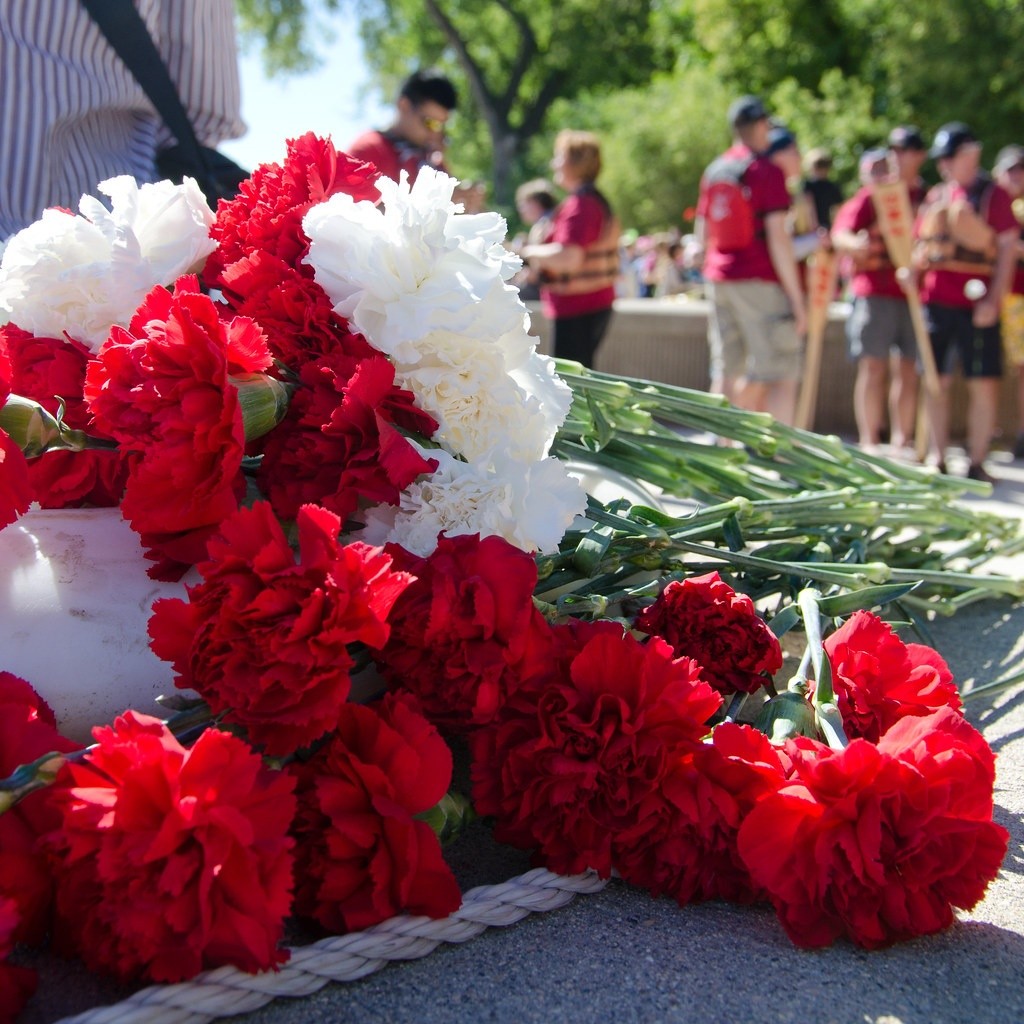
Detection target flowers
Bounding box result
[0,132,1024,1024]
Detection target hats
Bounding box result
[765,126,796,156]
[729,94,769,124]
[887,124,927,153]
[928,120,980,159]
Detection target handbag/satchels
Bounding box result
[101,0,251,213]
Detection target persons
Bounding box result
[348,69,483,214]
[0,0,250,261]
[830,127,930,460]
[692,98,846,428]
[910,125,1020,487]
[521,129,620,372]
[992,144,1024,467]
[504,178,699,300]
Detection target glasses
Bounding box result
[417,108,444,134]
